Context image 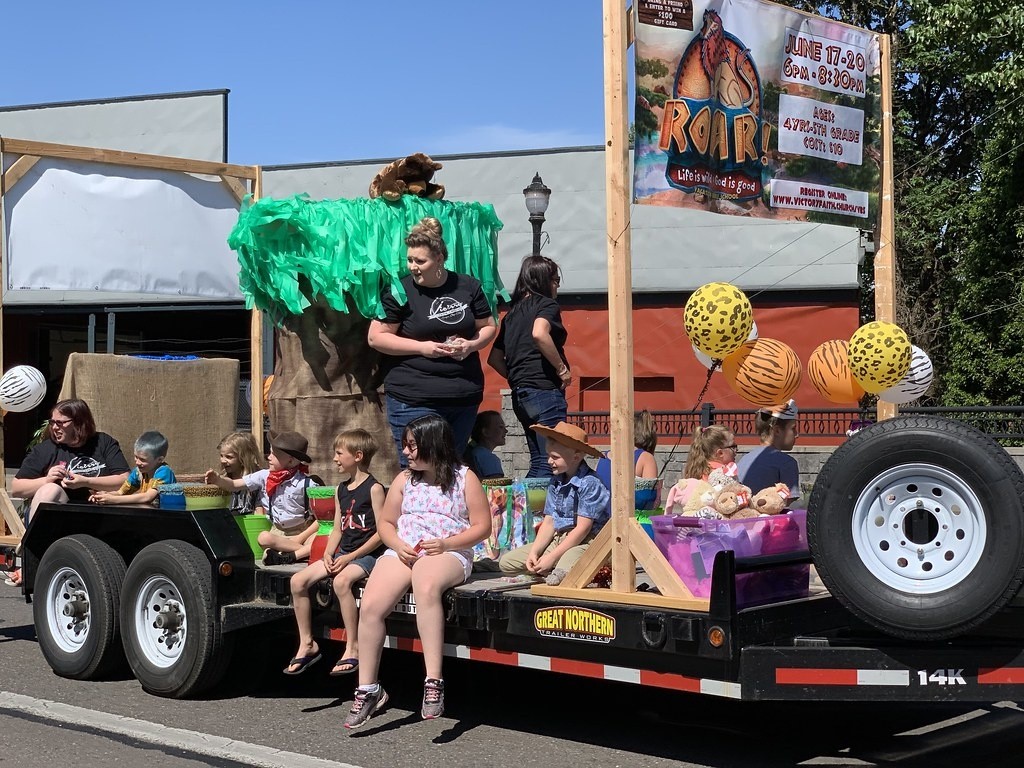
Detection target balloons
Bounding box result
[0,365,47,413]
[684,282,934,407]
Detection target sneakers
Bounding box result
[261,548,296,566]
[421,678,444,720]
[343,684,389,728]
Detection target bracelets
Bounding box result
[556,365,568,376]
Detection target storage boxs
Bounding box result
[651,509,812,608]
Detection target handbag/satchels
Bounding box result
[472,483,537,573]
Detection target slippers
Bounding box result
[284,652,322,674]
[0,570,22,587]
[329,658,359,676]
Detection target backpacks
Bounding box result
[268,475,326,522]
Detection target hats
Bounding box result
[756,399,798,419]
[267,430,312,463]
[529,421,605,459]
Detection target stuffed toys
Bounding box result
[369,152,446,201]
[664,462,809,610]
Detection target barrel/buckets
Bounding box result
[233,514,273,559]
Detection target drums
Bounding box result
[635,478,664,511]
[157,482,205,507]
[182,484,233,509]
[521,477,553,513]
[307,487,342,566]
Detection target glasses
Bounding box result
[552,276,560,283]
[49,419,73,427]
[716,442,737,453]
[402,440,417,451]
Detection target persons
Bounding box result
[685,424,739,482]
[342,414,492,729]
[487,255,573,478]
[499,421,610,577]
[368,217,508,478]
[4,399,132,587]
[594,410,663,539]
[737,399,800,515]
[88,430,186,505]
[205,427,319,566]
[283,428,386,675]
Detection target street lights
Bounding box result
[524,173,552,258]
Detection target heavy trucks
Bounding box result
[0,123,1024,764]
